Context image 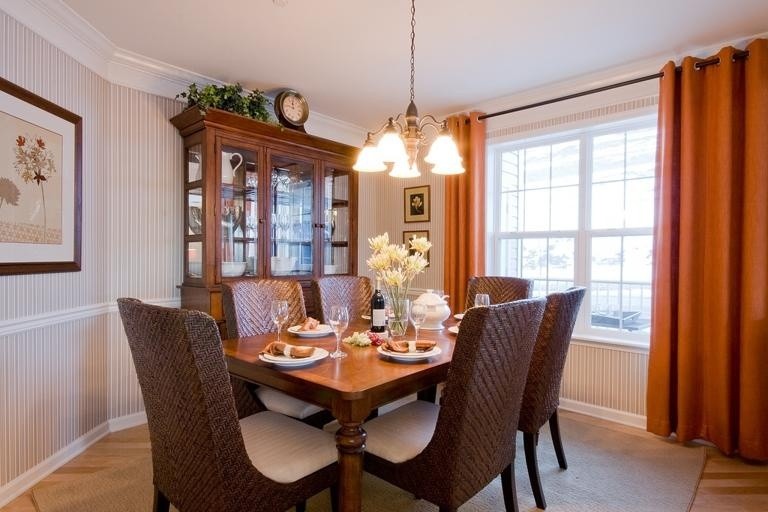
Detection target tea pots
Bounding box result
[409,287,451,331]
[193,153,204,179]
[222,151,243,183]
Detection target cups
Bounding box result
[471,293,490,309]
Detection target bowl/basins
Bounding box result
[220,261,248,278]
[323,264,339,275]
[271,256,299,276]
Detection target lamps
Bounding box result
[352,0,467,179]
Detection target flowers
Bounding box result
[365,232,434,334]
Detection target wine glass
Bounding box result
[246,211,311,240]
[270,170,304,194]
[269,299,290,342]
[329,306,349,359]
[411,302,428,341]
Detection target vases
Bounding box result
[383,294,410,342]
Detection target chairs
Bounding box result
[464,277,533,316]
[313,277,374,328]
[114,298,340,512]
[361,296,547,511]
[516,287,587,509]
[180,282,323,340]
[220,278,378,433]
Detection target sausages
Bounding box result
[274,341,316,359]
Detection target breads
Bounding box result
[299,316,320,331]
[381,337,437,353]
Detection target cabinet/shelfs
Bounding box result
[169,104,363,288]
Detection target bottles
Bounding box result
[369,275,386,331]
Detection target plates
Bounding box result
[361,315,389,322]
[446,314,466,335]
[375,340,443,363]
[285,323,336,338]
[258,344,329,367]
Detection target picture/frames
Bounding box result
[1,74,84,269]
[402,230,432,271]
[404,184,432,223]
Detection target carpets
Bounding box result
[31,415,710,511]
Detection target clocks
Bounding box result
[275,90,310,134]
